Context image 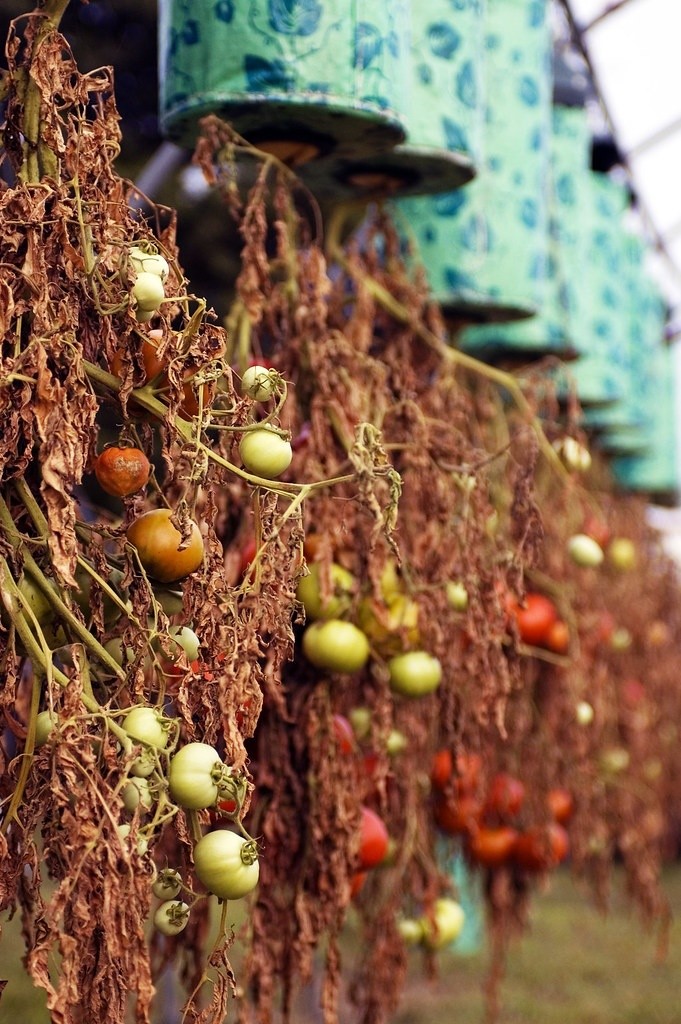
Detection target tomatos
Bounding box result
[33,238,636,951]
